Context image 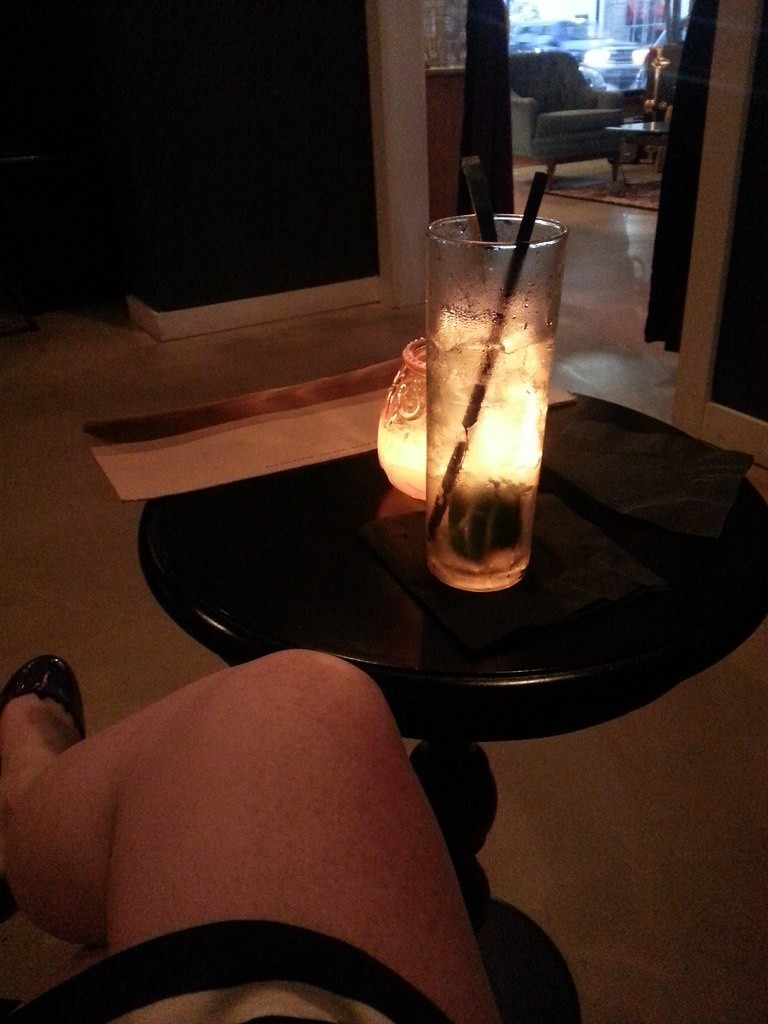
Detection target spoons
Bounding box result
[449,156,519,557]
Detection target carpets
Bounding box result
[541,161,660,211]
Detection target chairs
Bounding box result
[506,50,624,187]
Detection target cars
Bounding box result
[564,39,649,87]
[508,20,577,50]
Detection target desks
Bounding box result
[604,122,669,183]
[139,392,768,1024]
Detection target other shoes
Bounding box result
[0,654,85,923]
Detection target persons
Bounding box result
[0,649,500,1024]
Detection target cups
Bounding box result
[427,214,568,592]
[378,337,431,500]
